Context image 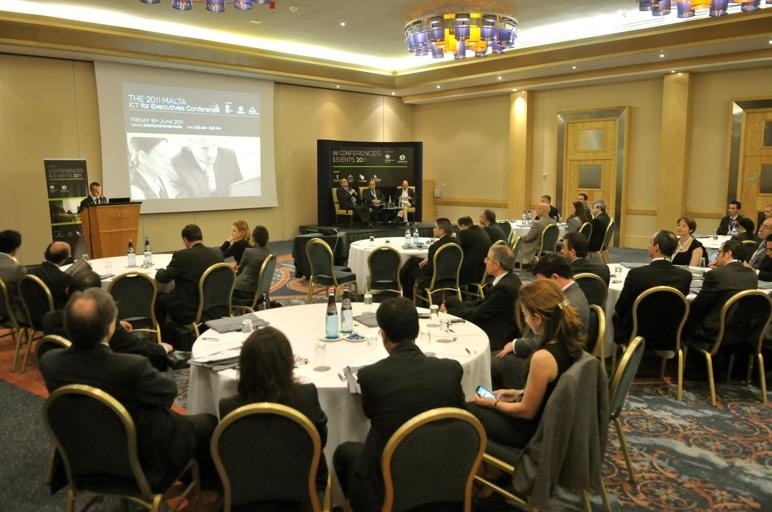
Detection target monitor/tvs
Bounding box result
[109,197,130,203]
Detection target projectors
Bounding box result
[319,227,340,235]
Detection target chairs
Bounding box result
[358,187,369,207]
[377,408,487,510]
[396,186,417,212]
[2,218,285,374]
[604,336,646,508]
[619,286,690,402]
[46,385,205,511]
[331,188,355,228]
[694,291,771,409]
[288,217,772,348]
[209,404,324,511]
[472,354,612,512]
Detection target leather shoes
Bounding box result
[166,350,191,370]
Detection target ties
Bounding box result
[95,198,99,205]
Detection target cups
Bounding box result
[313,343,331,371]
[362,293,374,311]
[241,318,253,343]
[428,305,438,320]
[102,260,112,275]
[612,266,623,284]
[368,235,391,248]
[438,318,449,337]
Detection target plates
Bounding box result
[319,332,342,342]
[346,332,368,342]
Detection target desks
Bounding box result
[182,301,495,499]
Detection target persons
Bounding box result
[394,181,416,225]
[128,137,178,200]
[38,287,219,506]
[1,217,276,371]
[408,193,771,388]
[337,178,376,229]
[220,327,329,512]
[334,299,466,512]
[80,182,107,212]
[363,180,385,222]
[467,278,584,502]
[169,135,243,198]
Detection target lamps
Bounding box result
[142,0,255,13]
[403,4,520,60]
[631,1,772,20]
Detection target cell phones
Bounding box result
[475,385,496,400]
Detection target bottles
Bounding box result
[144,236,152,267]
[339,284,356,333]
[405,221,419,247]
[522,209,532,225]
[325,288,338,338]
[127,237,135,267]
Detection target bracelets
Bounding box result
[493,398,500,408]
